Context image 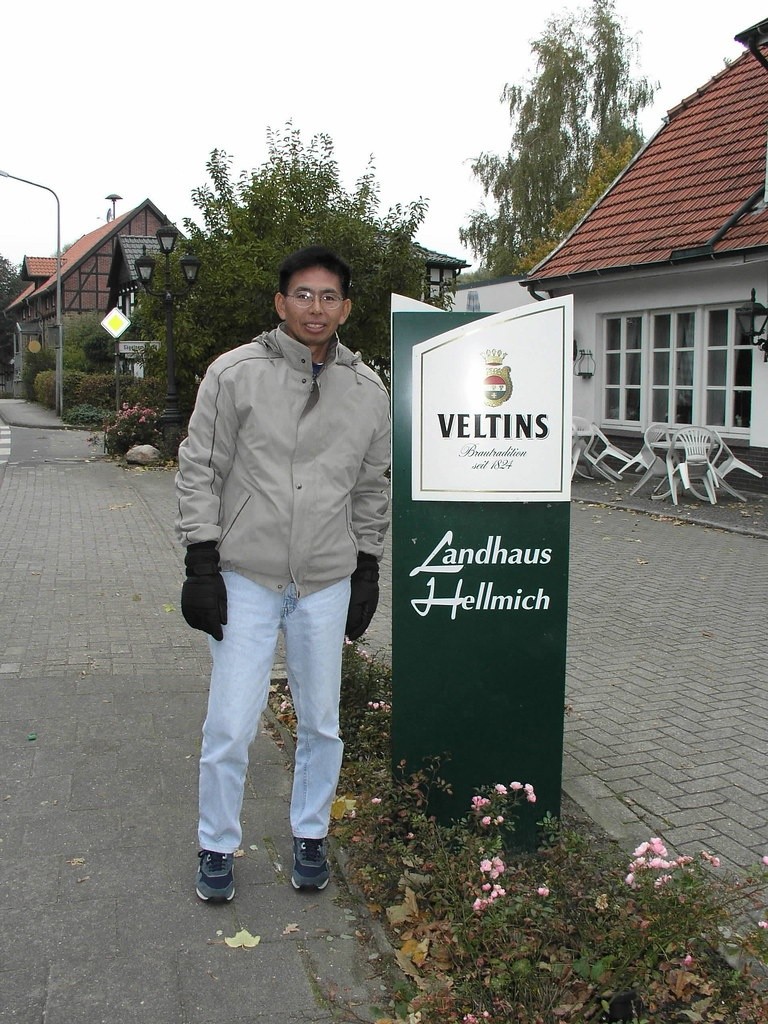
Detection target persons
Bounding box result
[173,247,394,906]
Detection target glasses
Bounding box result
[285,291,345,309]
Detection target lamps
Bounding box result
[736,288,768,362]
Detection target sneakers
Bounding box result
[195,849,236,902]
[291,836,331,889]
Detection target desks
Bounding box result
[572,431,598,480]
[649,441,719,502]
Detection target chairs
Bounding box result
[571,417,763,506]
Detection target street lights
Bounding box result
[0,170,63,415]
[134,214,201,453]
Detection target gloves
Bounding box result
[181,540,227,643]
[346,551,379,642]
